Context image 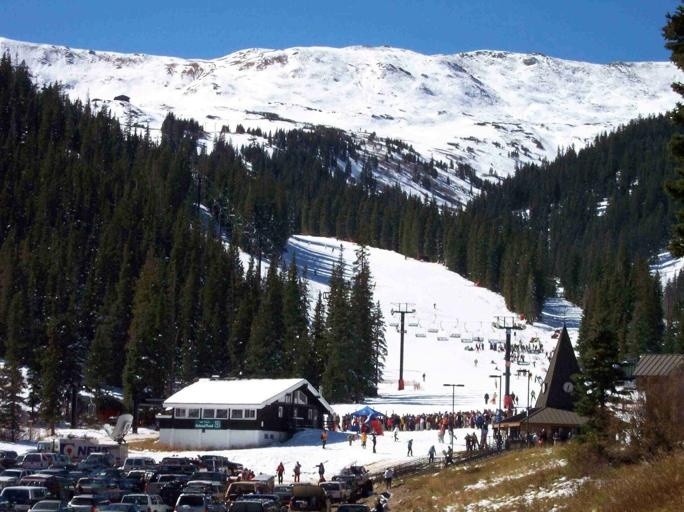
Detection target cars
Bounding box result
[1,449,372,512]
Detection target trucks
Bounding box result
[36,413,134,468]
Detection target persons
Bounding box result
[316,406,499,489]
[276,462,284,483]
[413,381,420,390]
[248,469,251,475]
[475,337,549,408]
[374,497,384,512]
[434,303,436,308]
[286,495,301,512]
[293,461,300,481]
[248,471,254,479]
[243,468,247,479]
[494,429,562,453]
[346,492,356,504]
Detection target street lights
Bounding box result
[444,369,532,453]
